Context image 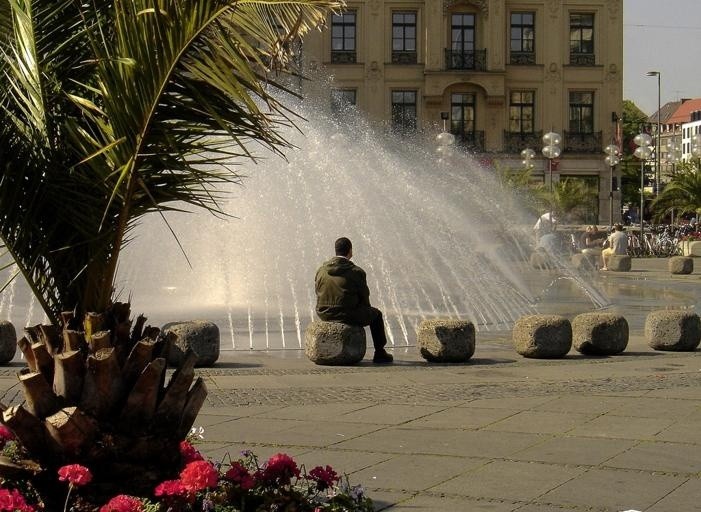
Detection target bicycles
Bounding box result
[564,225,582,253]
[599,217,700,257]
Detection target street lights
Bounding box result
[435,117,456,180]
[541,122,563,236]
[645,70,664,223]
[519,146,537,216]
[635,122,654,237]
[602,135,623,237]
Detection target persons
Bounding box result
[315,237,393,363]
[533,211,556,239]
[599,224,628,271]
[580,224,603,248]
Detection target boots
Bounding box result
[372,349,393,363]
